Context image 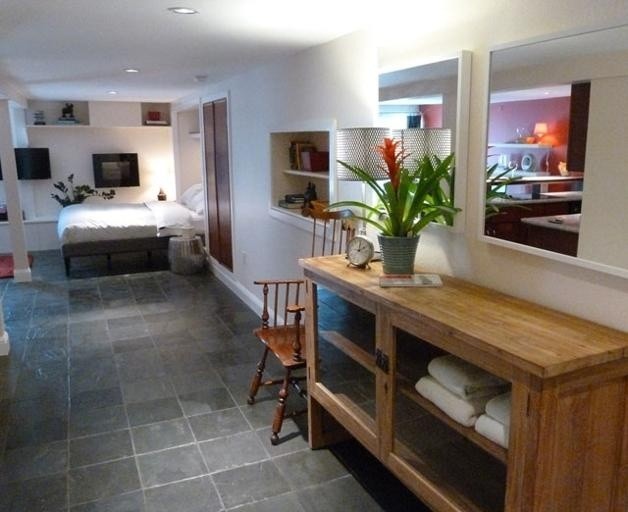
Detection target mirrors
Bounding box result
[479,16,628,280]
[375,48,473,233]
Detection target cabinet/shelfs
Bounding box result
[489,142,584,258]
[269,117,337,236]
[299,252,626,512]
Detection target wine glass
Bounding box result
[516,127,524,138]
[508,160,519,179]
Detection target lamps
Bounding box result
[331,126,389,247]
[534,122,548,143]
[396,126,451,178]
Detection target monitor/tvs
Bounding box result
[15,147,51,180]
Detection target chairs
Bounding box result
[248,217,365,443]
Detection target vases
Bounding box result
[372,229,422,276]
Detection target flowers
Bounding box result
[328,134,456,239]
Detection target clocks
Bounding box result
[344,236,374,267]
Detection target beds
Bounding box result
[56,198,206,279]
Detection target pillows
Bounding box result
[178,182,207,215]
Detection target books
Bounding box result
[56,120,80,124]
[279,199,306,209]
[287,139,329,172]
[59,117,75,121]
[285,193,306,204]
[145,120,168,126]
[378,273,443,288]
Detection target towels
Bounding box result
[415,355,513,452]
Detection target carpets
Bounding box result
[1,254,32,278]
